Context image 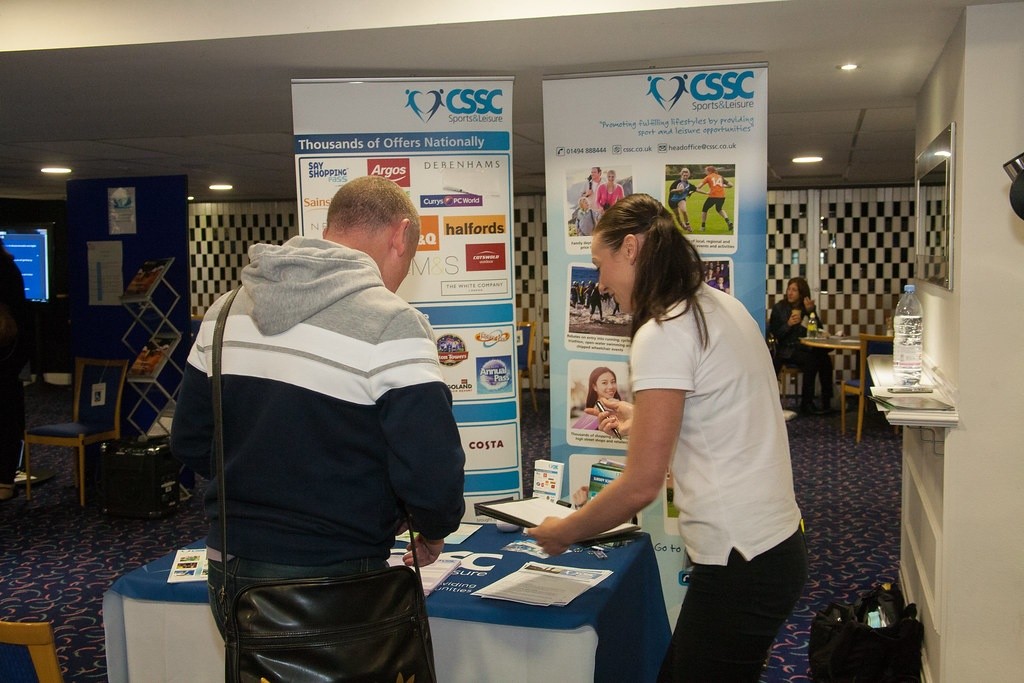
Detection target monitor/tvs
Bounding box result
[0,222,55,304]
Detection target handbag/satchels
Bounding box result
[225,566,437,683]
[808,583,924,683]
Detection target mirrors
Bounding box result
[914,122,957,293]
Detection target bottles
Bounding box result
[892,285,923,385]
[807,312,817,339]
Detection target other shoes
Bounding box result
[686,222,691,230]
[729,221,733,230]
[680,222,686,229]
[701,226,705,231]
[801,400,824,414]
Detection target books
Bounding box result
[127,337,177,376]
[122,257,176,298]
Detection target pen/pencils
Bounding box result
[595,401,622,441]
[887,387,933,393]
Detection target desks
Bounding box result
[800,336,861,351]
[103,523,672,683]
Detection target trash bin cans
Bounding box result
[100,437,180,518]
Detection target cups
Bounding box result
[791,308,802,319]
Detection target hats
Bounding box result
[573,280,595,286]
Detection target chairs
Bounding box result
[769,331,803,411]
[841,334,899,443]
[24,359,129,508]
[0,621,63,683]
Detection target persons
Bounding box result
[697,166,733,230]
[700,261,730,293]
[583,167,604,211]
[576,197,600,236]
[169,175,467,647]
[571,280,620,321]
[528,193,807,683]
[596,170,625,212]
[94,392,101,402]
[574,367,622,430]
[769,277,835,415]
[0,244,25,500]
[668,168,707,232]
